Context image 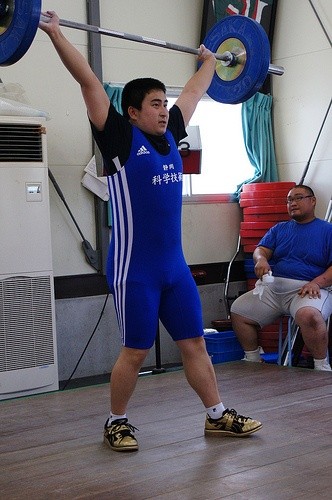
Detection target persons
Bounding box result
[39,9,264,451]
[231,185,332,373]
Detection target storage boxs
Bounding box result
[203,330,246,365]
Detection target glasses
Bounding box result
[286,195,312,204]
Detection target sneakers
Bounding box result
[203,408,264,438]
[102,417,140,453]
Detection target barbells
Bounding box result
[0,0,285,105]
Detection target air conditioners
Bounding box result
[0,115,60,401]
